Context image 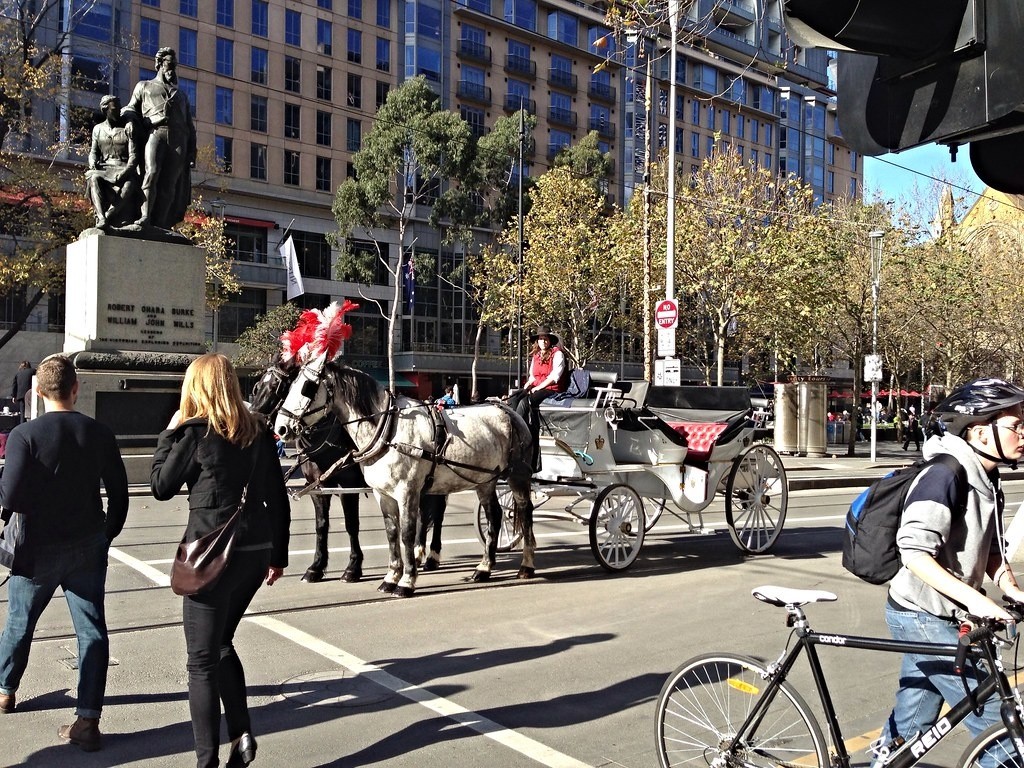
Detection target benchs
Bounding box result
[598,380,690,464]
[541,370,617,413]
[642,384,754,446]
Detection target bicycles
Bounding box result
[653,584,1023,768]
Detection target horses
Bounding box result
[248,357,449,583]
[273,345,536,597]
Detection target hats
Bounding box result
[529,325,559,346]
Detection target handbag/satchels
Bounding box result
[171,510,241,597]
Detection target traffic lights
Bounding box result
[783,1,1024,157]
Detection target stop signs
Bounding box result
[651,300,678,328]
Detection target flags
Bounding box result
[401,244,416,312]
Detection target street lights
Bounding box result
[869,229,886,463]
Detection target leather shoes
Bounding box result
[0,694,15,712]
[58,717,102,750]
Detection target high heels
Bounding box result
[226,733,257,768]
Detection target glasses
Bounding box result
[967,421,1024,435]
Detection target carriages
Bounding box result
[245,343,789,598]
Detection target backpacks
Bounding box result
[842,454,1001,585]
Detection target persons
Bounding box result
[150,353,291,768]
[84,95,140,229]
[870,378,1024,768]
[11,360,36,424]
[498,325,565,480]
[425,386,485,410]
[124,46,196,231]
[826,400,943,451]
[0,355,129,753]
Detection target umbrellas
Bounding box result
[825,388,936,412]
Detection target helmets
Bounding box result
[932,377,1024,439]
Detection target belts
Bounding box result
[886,586,908,611]
